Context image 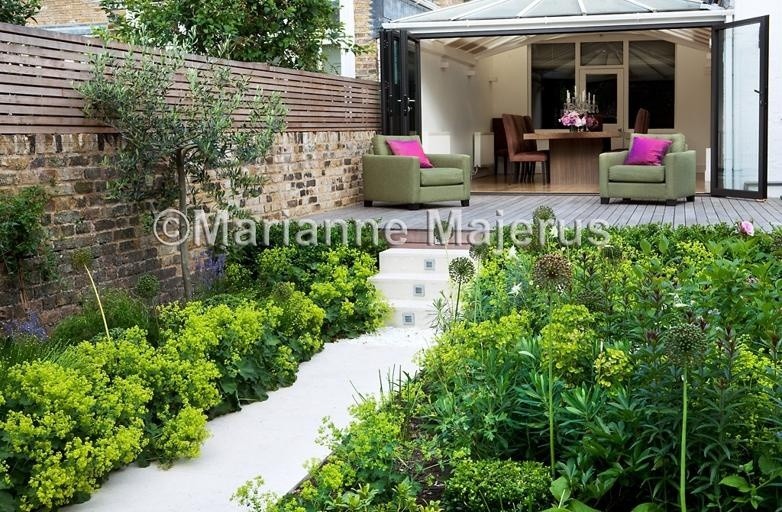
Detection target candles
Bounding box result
[565,85,596,105]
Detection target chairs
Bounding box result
[491,108,697,205]
[362,135,473,207]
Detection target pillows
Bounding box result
[383,139,433,168]
